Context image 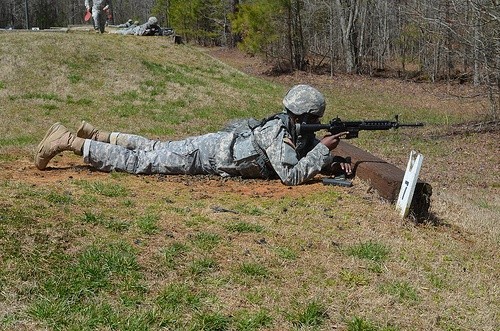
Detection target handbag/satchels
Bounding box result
[235,156,260,178]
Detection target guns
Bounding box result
[294,114,425,161]
[150,28,173,36]
[323,174,353,188]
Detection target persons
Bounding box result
[35,84,352,186]
[85,0,167,36]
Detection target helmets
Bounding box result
[283,84,326,117]
[134,21,139,25]
[128,19,132,23]
[148,16,158,25]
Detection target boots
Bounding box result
[34,122,85,170]
[74,120,111,155]
